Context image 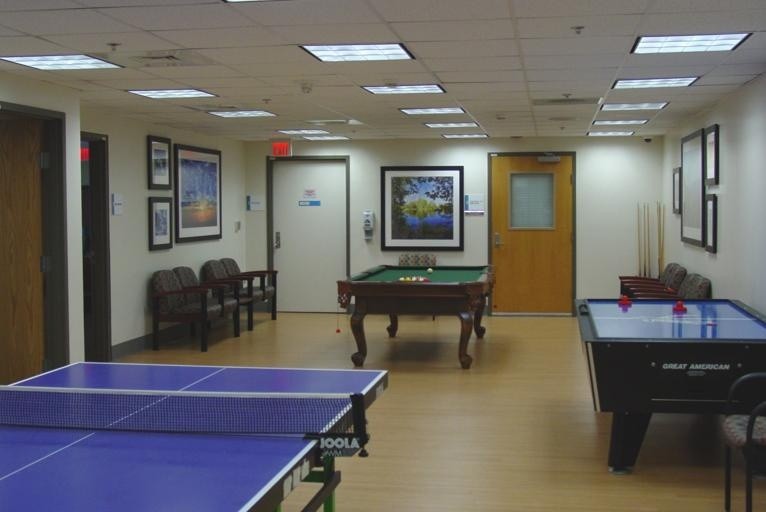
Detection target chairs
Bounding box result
[618,263,712,300]
[150,258,279,352]
[720,372,765,512]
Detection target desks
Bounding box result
[337,264,496,369]
[0,360,390,512]
[573,298,766,474]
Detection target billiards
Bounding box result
[399,275,431,282]
[427,268,433,273]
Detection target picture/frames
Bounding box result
[704,193,717,253]
[173,143,222,243]
[703,123,719,185]
[681,128,705,247]
[380,165,464,251]
[672,166,682,214]
[146,135,173,190]
[148,196,173,250]
